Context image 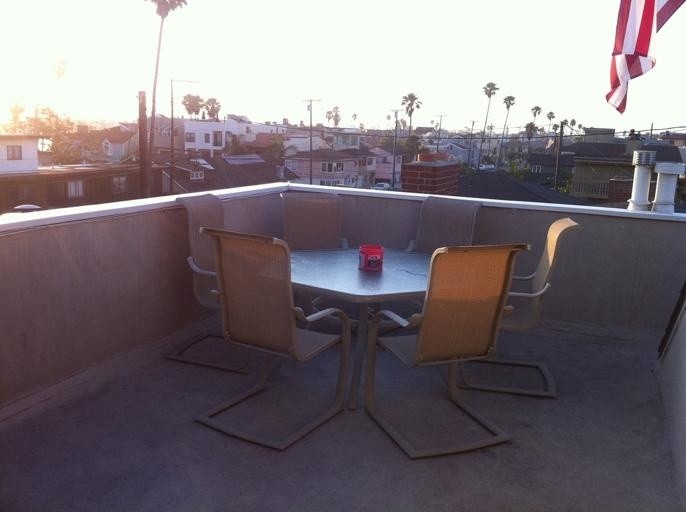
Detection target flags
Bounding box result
[606,0,686,114]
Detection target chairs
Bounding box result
[280,192,341,248]
[167,193,254,374]
[363,244,531,459]
[456,217,581,399]
[195,228,350,451]
[415,197,483,253]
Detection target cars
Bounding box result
[479,164,494,171]
[374,182,389,190]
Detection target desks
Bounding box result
[290,247,433,410]
